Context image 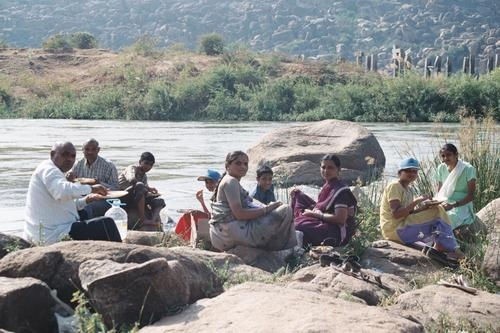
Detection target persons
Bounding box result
[64,137,119,219]
[251,165,276,206]
[289,154,357,252]
[431,142,477,251]
[196,169,221,213]
[380,157,470,263]
[118,152,166,227]
[207,151,298,251]
[23,140,123,246]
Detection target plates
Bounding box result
[71,177,96,184]
[149,191,161,197]
[425,199,444,205]
[177,208,195,213]
[95,190,129,199]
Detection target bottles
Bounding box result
[104,199,128,241]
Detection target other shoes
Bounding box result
[427,248,458,266]
[422,245,432,255]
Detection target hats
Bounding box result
[197,170,221,183]
[399,157,421,173]
